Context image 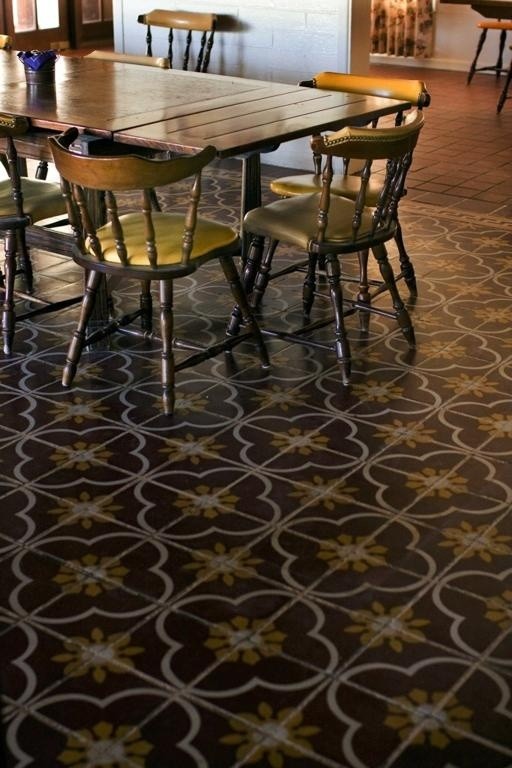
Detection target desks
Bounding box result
[2,50,410,280]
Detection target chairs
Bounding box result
[468,1,512,84]
[228,107,427,392]
[0,113,115,355]
[46,127,271,415]
[136,8,218,73]
[247,69,431,312]
